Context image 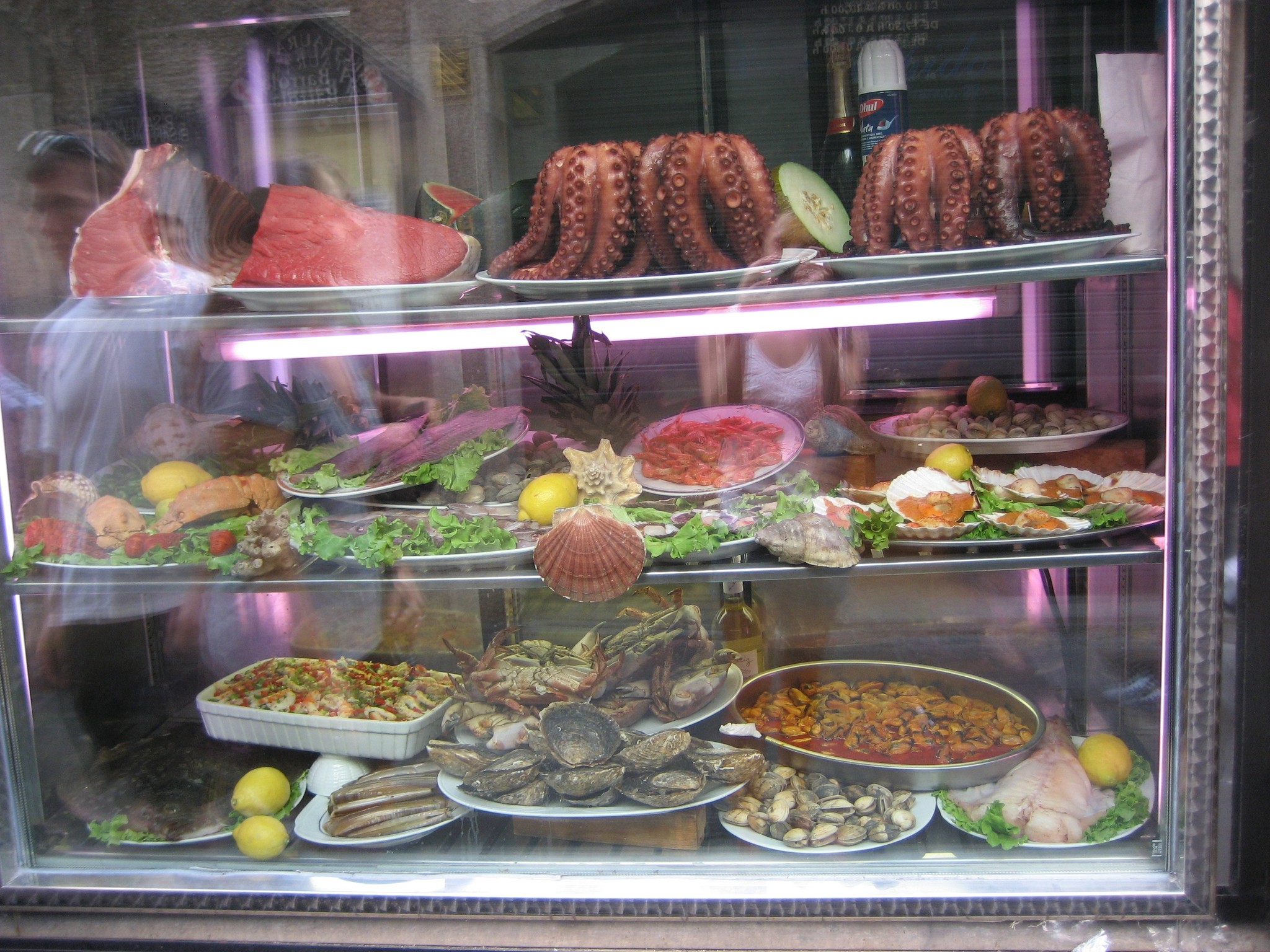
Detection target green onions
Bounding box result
[208,657,453,720]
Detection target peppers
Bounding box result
[94,453,273,505]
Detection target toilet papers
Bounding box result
[1094,51,1167,258]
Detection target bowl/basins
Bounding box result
[305,753,370,796]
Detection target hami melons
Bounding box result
[770,163,852,255]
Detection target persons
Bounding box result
[18,122,439,747]
[699,214,873,688]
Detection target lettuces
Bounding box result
[276,384,519,574]
[583,468,1131,559]
[932,748,1153,849]
[86,768,310,844]
[0,504,266,581]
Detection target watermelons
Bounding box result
[414,177,538,267]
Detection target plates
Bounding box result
[278,410,528,499]
[863,517,1163,546]
[333,512,541,569]
[90,458,160,516]
[622,405,807,496]
[870,408,1130,454]
[57,292,207,319]
[454,663,743,748]
[474,249,816,298]
[937,736,1157,846]
[436,743,751,819]
[36,558,204,568]
[731,659,1044,791]
[718,789,937,854]
[208,283,485,313]
[656,535,762,563]
[339,430,597,505]
[290,794,477,850]
[823,234,1143,279]
[88,779,304,846]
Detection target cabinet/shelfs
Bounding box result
[0,0,1248,922]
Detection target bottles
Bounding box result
[711,582,768,681]
[821,42,862,221]
[859,40,910,168]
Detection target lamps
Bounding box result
[201,285,1024,362]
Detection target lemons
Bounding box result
[231,767,290,816]
[922,444,973,480]
[966,375,1009,416]
[232,814,289,861]
[1077,733,1131,788]
[139,460,214,506]
[517,472,578,526]
